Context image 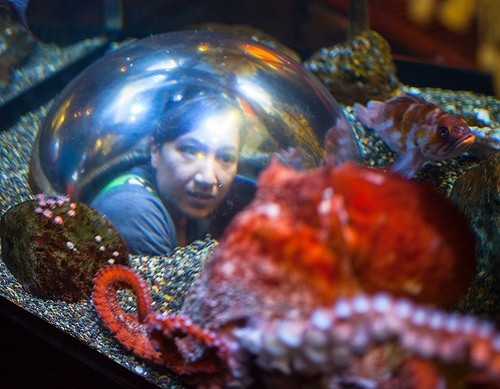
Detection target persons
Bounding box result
[81,80,261,261]
